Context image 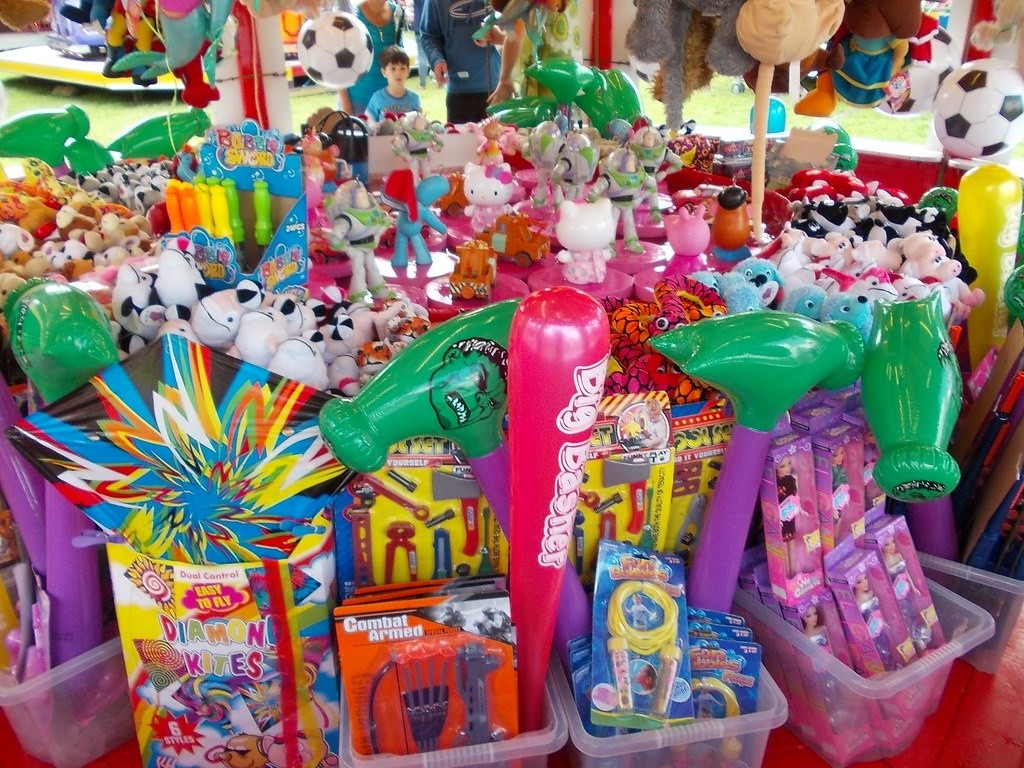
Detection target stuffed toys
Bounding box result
[0,1,986,403]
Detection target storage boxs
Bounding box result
[719,538,997,768]
[159,118,310,307]
[0,632,143,768]
[339,641,570,768]
[106,488,344,768]
[914,543,1024,675]
[550,624,792,768]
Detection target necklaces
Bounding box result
[370,10,384,42]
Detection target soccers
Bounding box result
[928,55,1024,161]
[293,9,373,89]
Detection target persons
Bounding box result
[412,0,443,89]
[364,42,423,134]
[419,0,502,126]
[476,0,583,109]
[337,0,407,116]
